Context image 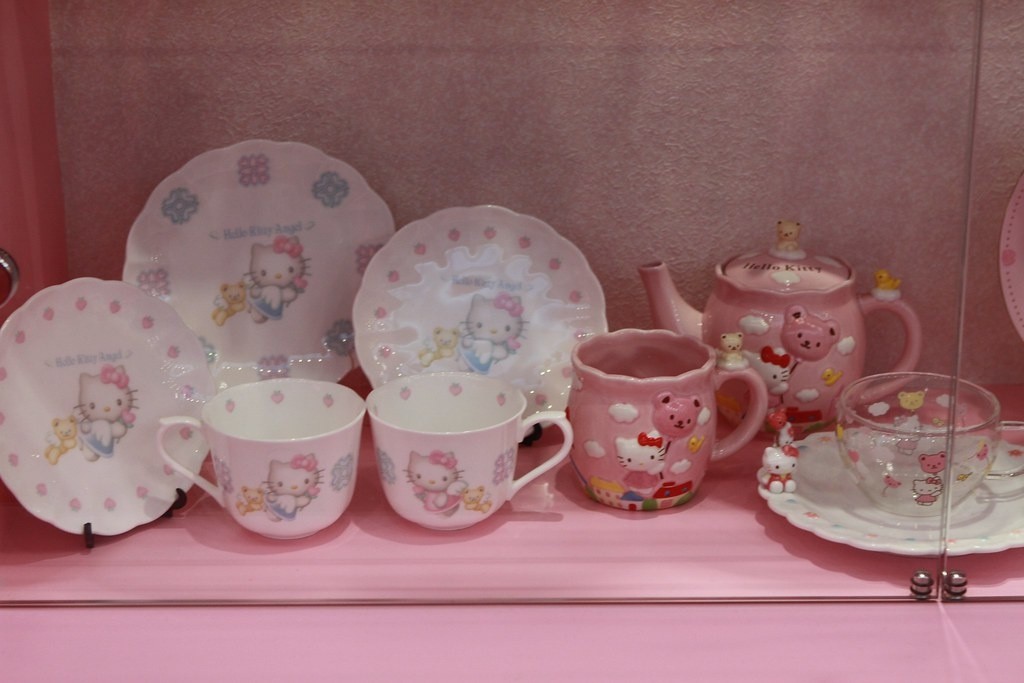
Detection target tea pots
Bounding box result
[638,219,923,437]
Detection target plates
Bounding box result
[757,424,1024,555]
[122,139,395,385]
[353,203,608,434]
[1,277,217,536]
[998,171,1024,338]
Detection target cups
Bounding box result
[157,378,366,540]
[366,372,575,530]
[564,328,769,511]
[835,373,1023,516]
